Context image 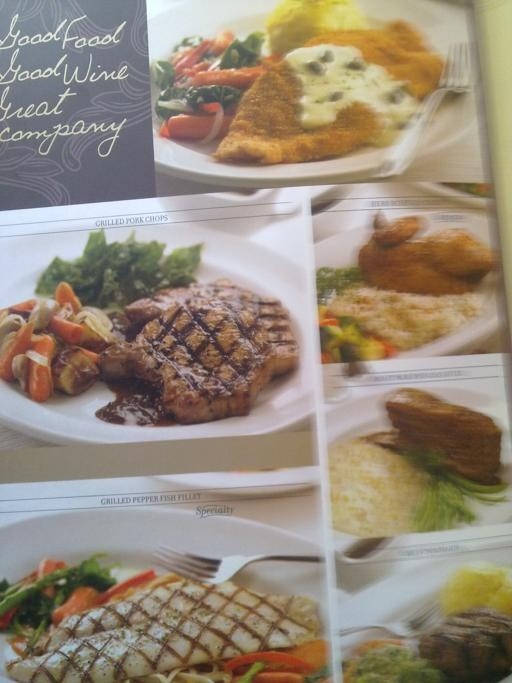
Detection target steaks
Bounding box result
[99,279,298,426]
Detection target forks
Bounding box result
[380,35,479,181]
[150,540,324,586]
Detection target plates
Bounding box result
[316,207,510,681]
[147,0,471,185]
[1,221,315,451]
[0,510,327,683]
